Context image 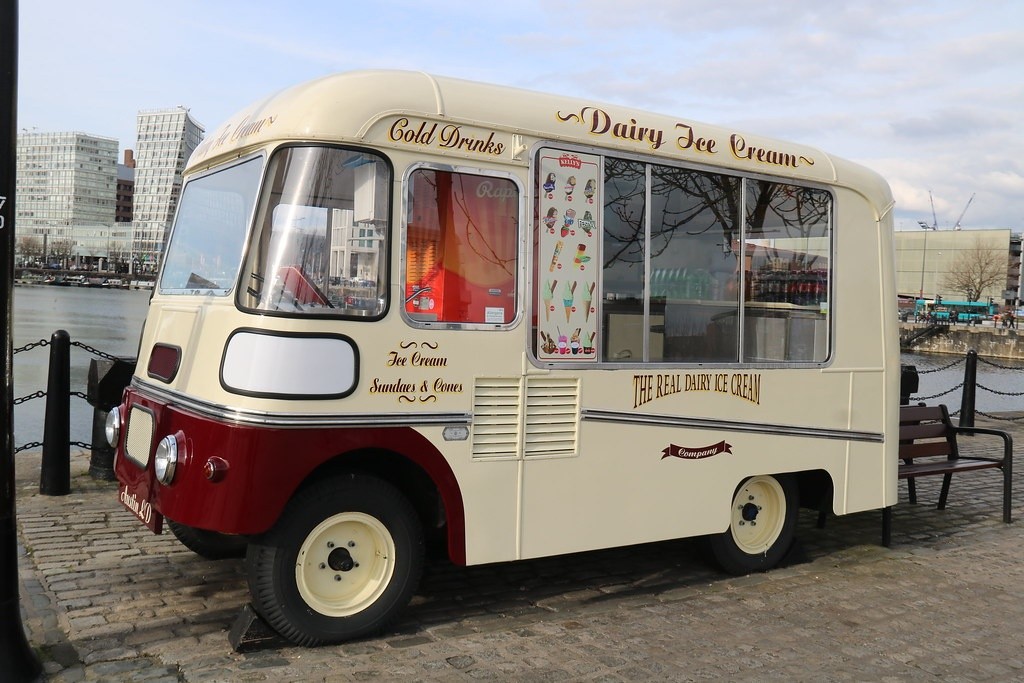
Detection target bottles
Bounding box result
[648,265,736,301]
[737,269,826,306]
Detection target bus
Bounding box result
[913,294,998,324]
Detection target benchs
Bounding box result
[900,401,927,504]
[817,404,1012,547]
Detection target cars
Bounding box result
[49,263,63,269]
[897,307,908,322]
[89,261,100,270]
[330,276,376,288]
[69,264,83,272]
[903,308,914,316]
[1011,309,1024,317]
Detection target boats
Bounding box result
[15,271,156,289]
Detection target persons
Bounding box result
[922,311,931,323]
[951,312,959,325]
[1002,313,1015,328]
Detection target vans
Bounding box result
[105,68,902,649]
